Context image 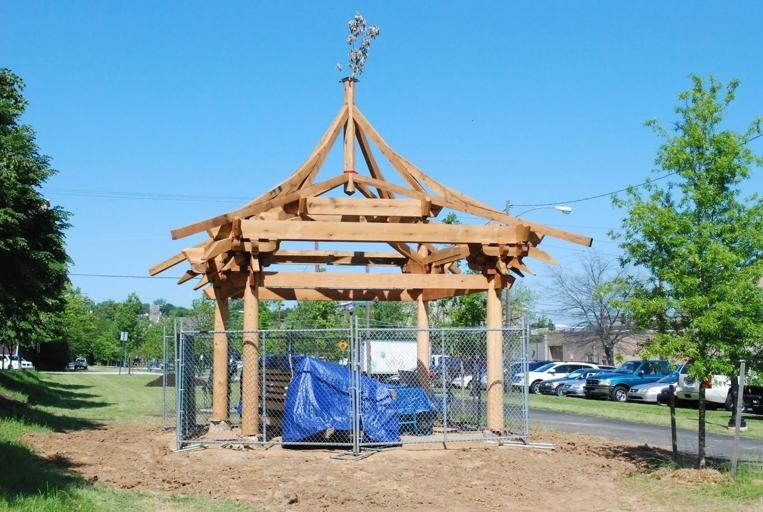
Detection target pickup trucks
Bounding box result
[74,358,87,369]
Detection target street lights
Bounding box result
[503,204,572,394]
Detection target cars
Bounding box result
[145,358,174,372]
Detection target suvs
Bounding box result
[0,353,33,371]
